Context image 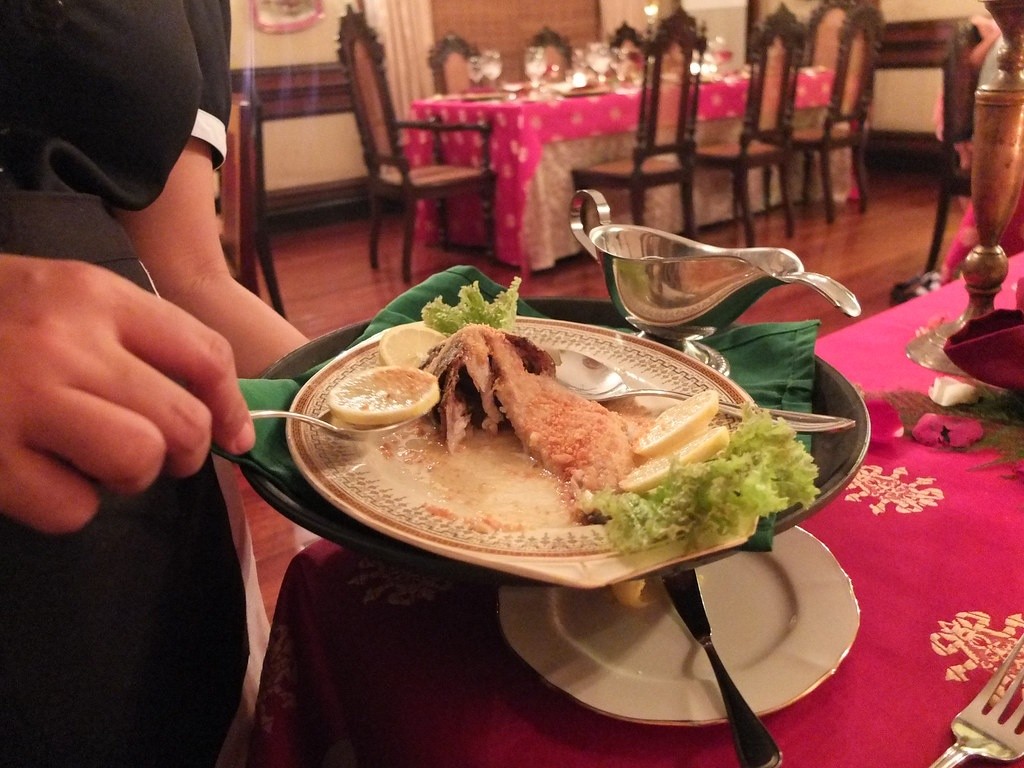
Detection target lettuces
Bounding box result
[421,275,523,337]
[576,400,822,557]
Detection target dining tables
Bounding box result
[243,249,1024,767]
[407,65,851,275]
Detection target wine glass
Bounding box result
[467,49,504,100]
[564,43,634,91]
[523,47,547,100]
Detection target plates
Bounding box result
[497,523,863,723]
[285,315,767,591]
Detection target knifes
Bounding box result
[661,569,785,768]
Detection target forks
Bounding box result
[928,633,1024,768]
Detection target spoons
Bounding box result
[243,409,425,439]
[551,350,858,433]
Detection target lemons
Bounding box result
[330,365,440,424]
[633,389,718,456]
[378,324,446,370]
[617,425,731,491]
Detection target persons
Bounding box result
[0,1,312,768]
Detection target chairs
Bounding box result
[426,31,485,95]
[220,100,288,317]
[522,23,572,82]
[336,7,498,281]
[759,0,887,226]
[690,3,808,250]
[605,15,645,57]
[572,7,708,266]
[887,20,1001,302]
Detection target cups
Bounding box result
[566,186,863,378]
